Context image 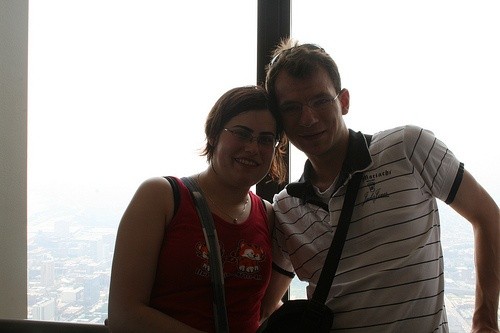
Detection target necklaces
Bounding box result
[198,174,248,222]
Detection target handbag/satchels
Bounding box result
[257,301,334,333]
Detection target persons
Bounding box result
[257,37,500,333]
[104,87,286,333]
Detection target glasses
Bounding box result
[278,92,339,117]
[224,128,277,146]
